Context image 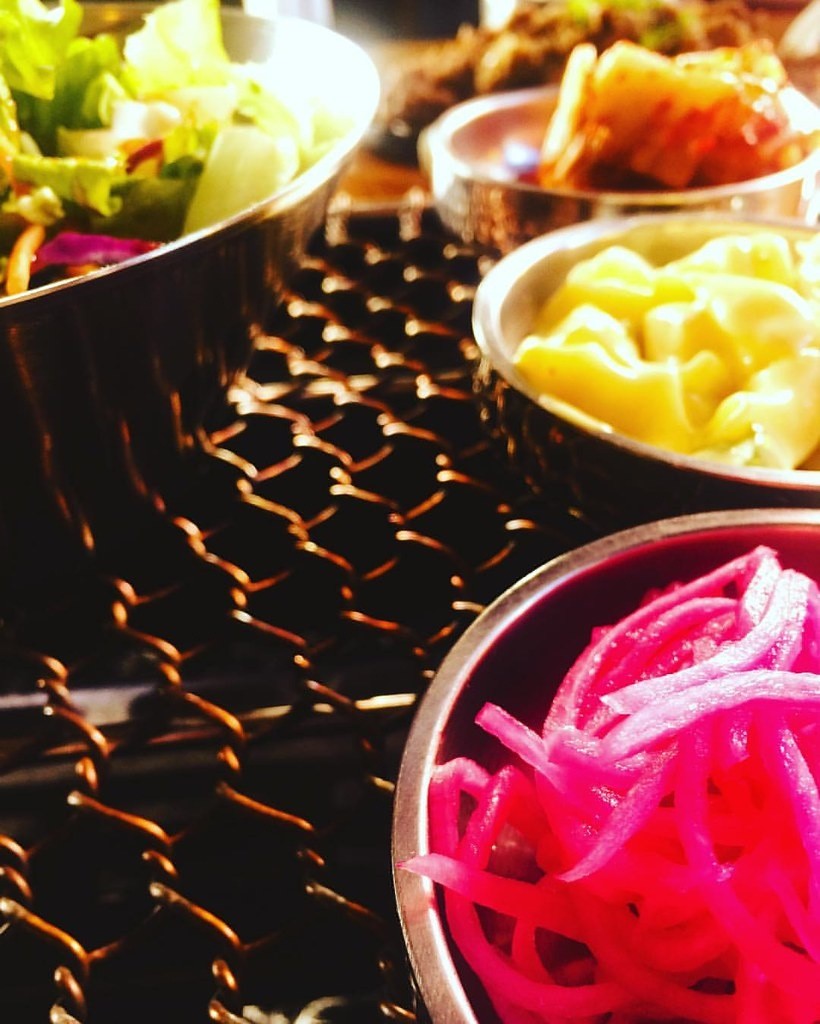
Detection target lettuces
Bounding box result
[0,0,342,297]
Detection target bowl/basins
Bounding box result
[427,86,819,265]
[391,508,820,1024]
[472,212,820,531]
[0,0,381,536]
